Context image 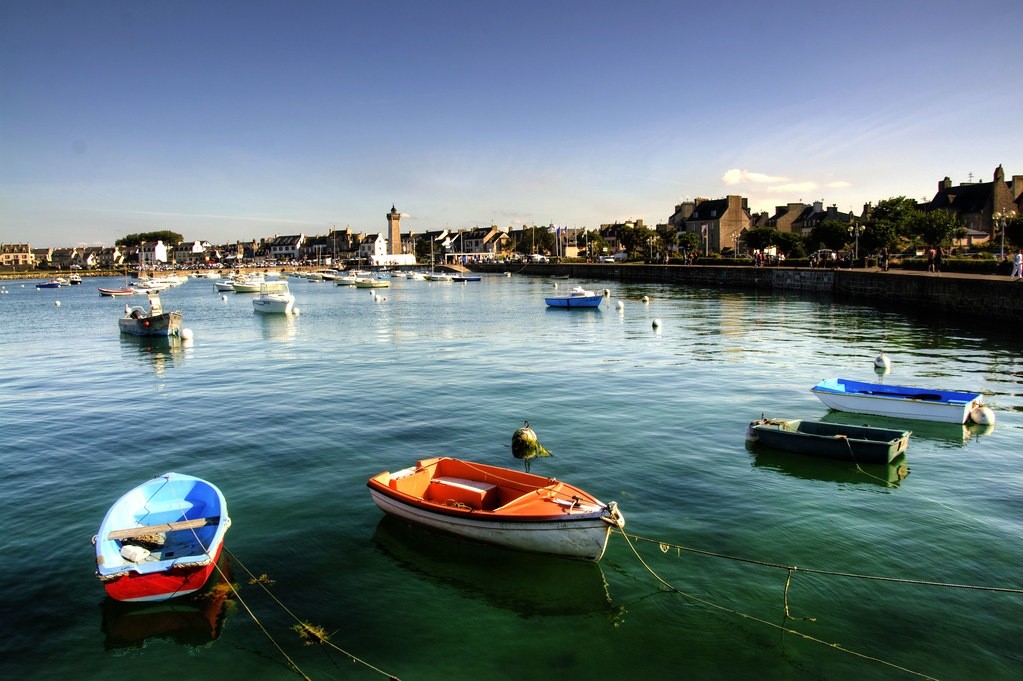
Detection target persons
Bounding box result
[684,251,697,266]
[830,250,839,269]
[1010,248,1023,279]
[926,245,943,273]
[809,250,827,269]
[754,250,785,267]
[877,247,890,272]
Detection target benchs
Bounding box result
[431,477,497,511]
[107,514,219,539]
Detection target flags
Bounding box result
[557,227,560,236]
[562,227,567,234]
[571,229,576,238]
[582,227,587,238]
[547,224,556,233]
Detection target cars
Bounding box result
[602,256,614,263]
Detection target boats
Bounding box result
[252,280,296,315]
[35,277,61,288]
[355,278,391,288]
[810,376,984,425]
[452,275,482,282]
[749,413,912,465]
[91,472,231,602]
[68,272,82,285]
[118,292,182,335]
[544,285,605,308]
[98,287,134,296]
[422,273,458,281]
[368,455,626,564]
[121,225,429,292]
[55,276,70,286]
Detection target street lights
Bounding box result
[729,230,741,259]
[847,222,866,258]
[992,207,1016,267]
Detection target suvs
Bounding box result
[525,254,549,264]
